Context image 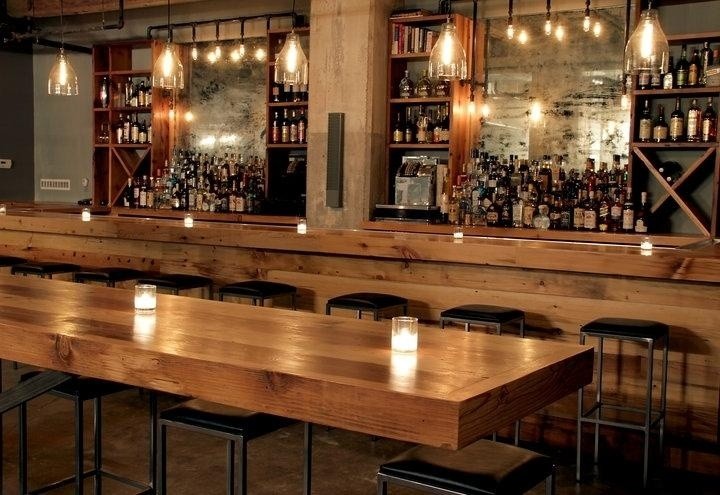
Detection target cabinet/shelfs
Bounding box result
[384,12,487,218]
[626,32,719,245]
[90,35,170,206]
[263,26,308,219]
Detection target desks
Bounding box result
[1,272,595,454]
[1,199,717,456]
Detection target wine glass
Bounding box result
[95,123,109,144]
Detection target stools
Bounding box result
[375,438,558,495]
[155,397,316,495]
[322,291,409,442]
[0,249,214,303]
[573,317,677,488]
[15,370,155,495]
[439,303,527,448]
[218,280,299,311]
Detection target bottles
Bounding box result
[440,149,650,234]
[639,96,718,143]
[636,41,720,91]
[270,106,309,144]
[122,145,265,212]
[393,102,449,143]
[116,112,151,144]
[399,69,450,98]
[98,76,153,108]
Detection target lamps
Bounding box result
[150,1,187,90]
[187,14,277,60]
[44,1,81,97]
[426,0,468,84]
[272,1,310,86]
[501,1,595,40]
[621,1,670,76]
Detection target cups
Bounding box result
[133,284,158,315]
[183,213,193,223]
[389,352,418,394]
[641,251,651,257]
[390,314,419,352]
[296,219,307,230]
[640,236,653,250]
[453,226,464,239]
[297,230,307,235]
[132,314,156,344]
[81,207,90,218]
[184,223,194,229]
[0,204,7,213]
[82,219,90,222]
